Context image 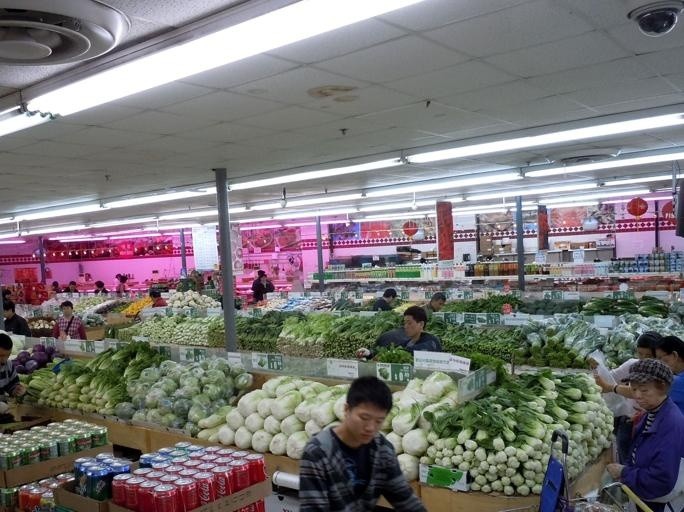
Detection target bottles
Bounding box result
[312,255,610,278]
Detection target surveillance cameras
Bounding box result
[628,0,684,38]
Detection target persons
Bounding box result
[589,331,663,464]
[355,284,446,361]
[0,274,131,339]
[607,358,684,512]
[149,288,168,307]
[298,375,429,512]
[0,333,28,424]
[253,274,273,300]
[596,335,684,416]
[251,269,275,291]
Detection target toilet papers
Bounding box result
[273,469,302,493]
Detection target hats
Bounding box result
[621,357,673,386]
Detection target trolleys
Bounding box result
[490,482,659,512]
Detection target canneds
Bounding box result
[0,419,265,512]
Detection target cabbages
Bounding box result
[114,359,351,460]
[379,371,459,482]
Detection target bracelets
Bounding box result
[612,384,620,394]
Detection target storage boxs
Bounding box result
[1,446,274,512]
[416,461,471,494]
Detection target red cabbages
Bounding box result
[10,344,65,373]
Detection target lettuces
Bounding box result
[38,340,165,415]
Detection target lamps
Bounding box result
[227,113,683,232]
[1,188,218,242]
[24,0,460,119]
